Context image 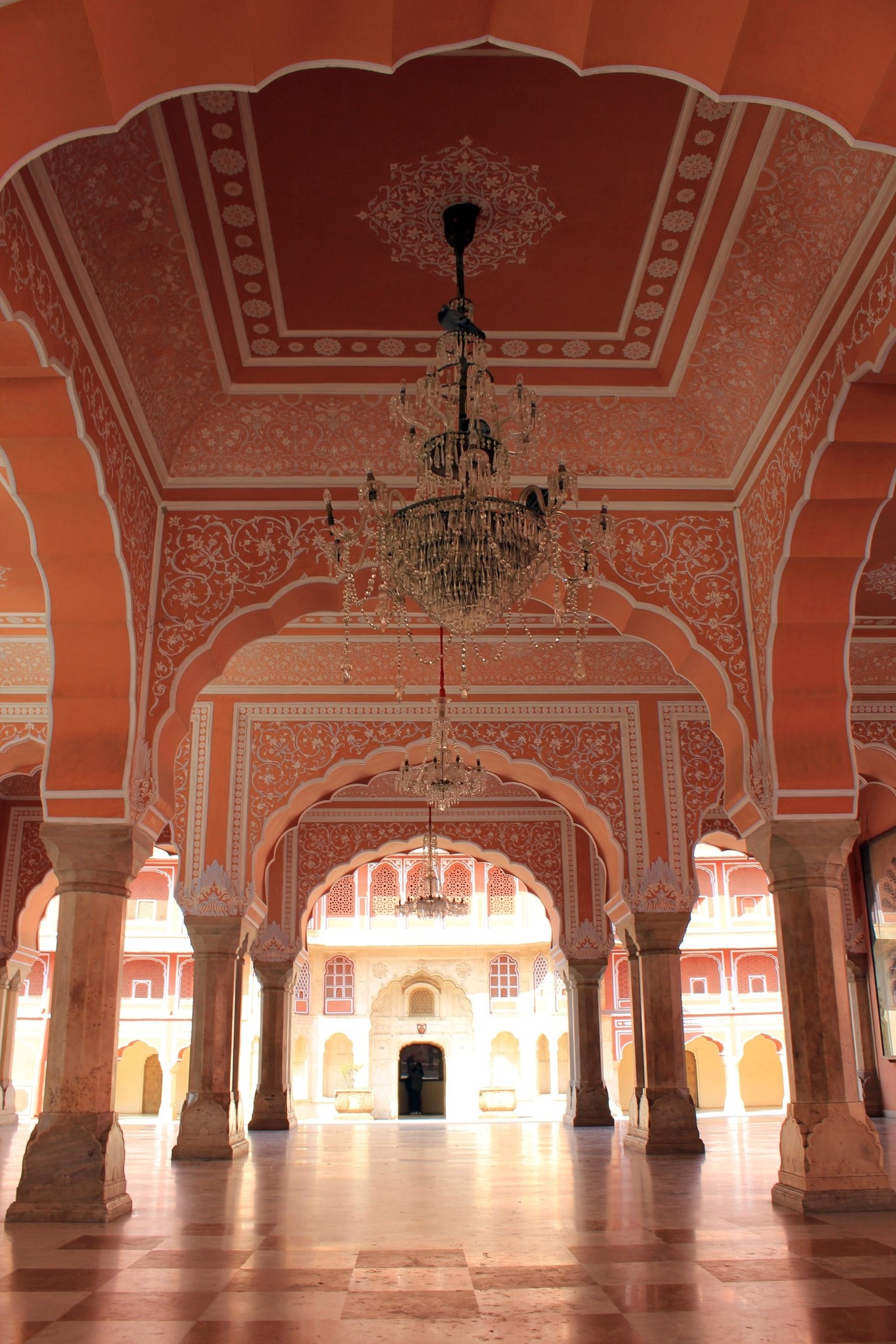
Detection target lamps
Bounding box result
[396,807,467,922]
[324,202,612,704]
[395,624,487,814]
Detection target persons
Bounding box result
[406,1056,423,1114]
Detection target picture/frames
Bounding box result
[859,825,896,1060]
